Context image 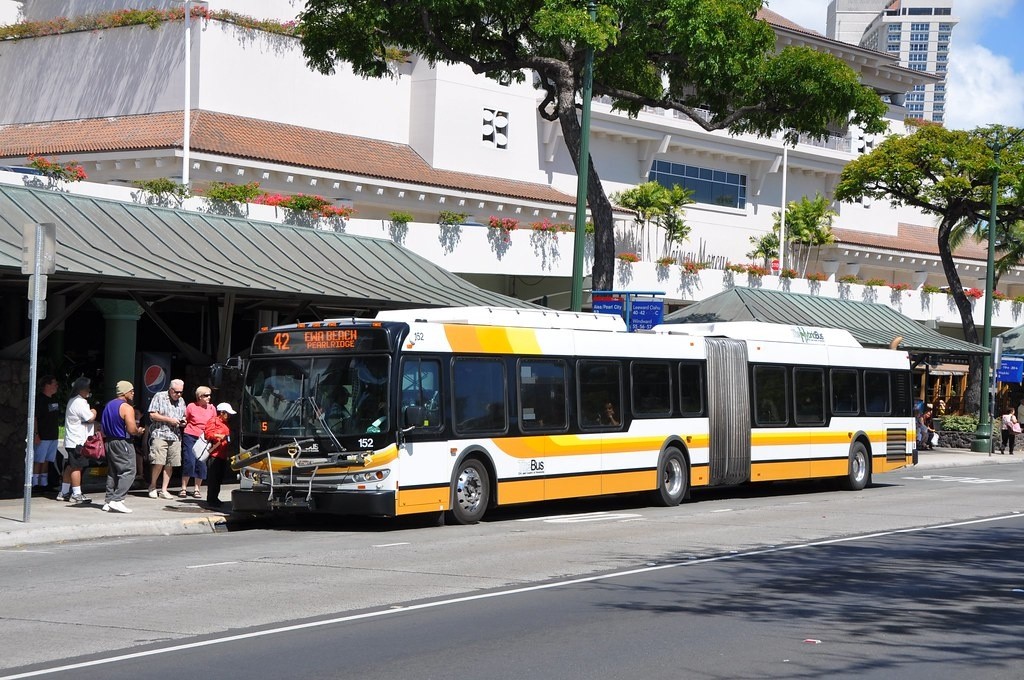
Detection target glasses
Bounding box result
[171,388,183,394]
[198,394,212,399]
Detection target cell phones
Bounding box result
[179,419,185,423]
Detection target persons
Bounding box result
[1017,399,1024,433]
[148,379,186,500]
[178,386,217,497]
[947,391,959,412]
[1000,408,1018,454]
[32,375,59,496]
[917,410,936,450]
[101,380,144,513]
[57,377,97,504]
[204,403,237,508]
[133,406,149,480]
[595,401,620,425]
[332,387,352,417]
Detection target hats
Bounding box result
[332,387,353,397]
[217,402,237,415]
[116,380,134,397]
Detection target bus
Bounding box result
[209,305,918,527]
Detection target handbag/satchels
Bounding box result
[79,431,107,459]
[931,432,940,446]
[1005,415,1021,435]
[192,432,213,463]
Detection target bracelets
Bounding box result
[35,432,38,436]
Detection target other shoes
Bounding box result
[193,490,202,498]
[1000,447,1005,454]
[178,489,187,498]
[1009,452,1014,455]
[159,491,174,500]
[148,486,157,498]
[31,485,51,497]
[208,498,224,507]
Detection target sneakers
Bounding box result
[102,503,116,512]
[108,501,132,513]
[69,493,92,504]
[56,492,71,501]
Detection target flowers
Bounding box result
[191,180,359,221]
[656,257,1024,303]
[616,252,641,266]
[29,153,87,183]
[488,216,573,245]
